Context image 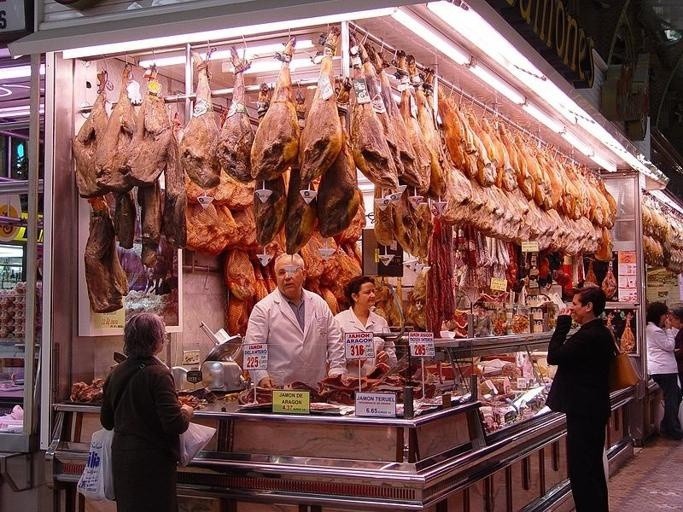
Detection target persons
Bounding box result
[544,284,616,512]
[246,252,348,393]
[331,275,399,383]
[645,300,683,438]
[100,312,194,510]
[668,303,682,388]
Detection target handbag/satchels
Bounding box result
[609,353,642,394]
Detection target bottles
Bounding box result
[1,258,21,287]
[600,261,616,300]
[583,258,598,285]
[561,256,573,290]
[604,312,614,346]
[619,312,637,352]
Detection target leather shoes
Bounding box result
[660,431,682,437]
